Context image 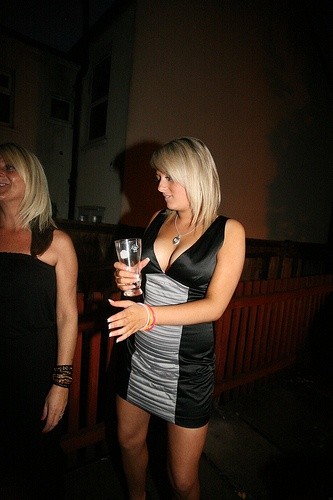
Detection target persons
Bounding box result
[107,136,247,500]
[0,139,81,500]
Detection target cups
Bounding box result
[114,238,144,297]
[78,205,105,223]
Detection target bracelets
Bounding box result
[137,302,156,332]
[52,364,74,388]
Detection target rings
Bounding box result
[59,415,63,419]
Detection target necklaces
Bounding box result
[172,213,204,245]
